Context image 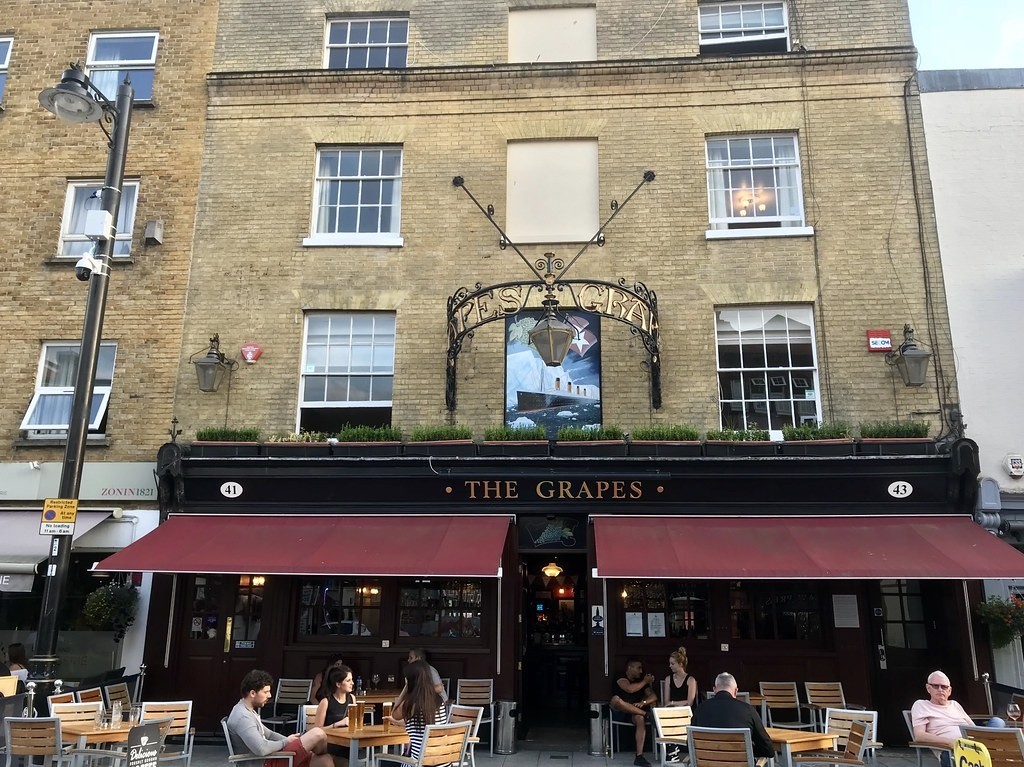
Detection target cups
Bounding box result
[348,704,357,732]
[111,701,123,729]
[383,701,392,731]
[356,700,365,729]
[95,711,107,730]
[129,708,140,726]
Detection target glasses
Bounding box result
[929,684,949,690]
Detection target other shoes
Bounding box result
[667,745,680,756]
[666,755,680,763]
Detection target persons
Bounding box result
[313,665,366,761]
[0,663,28,709]
[406,647,449,704]
[910,671,976,767]
[664,648,699,762]
[227,668,334,767]
[691,673,776,759]
[604,660,657,767]
[445,606,480,636]
[381,659,455,767]
[6,641,30,684]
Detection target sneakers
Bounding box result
[644,712,654,726]
[634,757,652,767]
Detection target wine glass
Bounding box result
[1006,704,1021,728]
[372,674,380,692]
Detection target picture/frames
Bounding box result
[556,598,575,624]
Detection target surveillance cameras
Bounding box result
[75,259,92,281]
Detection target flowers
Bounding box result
[975,594,1024,650]
[81,578,139,644]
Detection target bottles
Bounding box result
[356,676,362,694]
[423,588,481,607]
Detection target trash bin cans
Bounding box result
[588,702,611,756]
[493,700,517,753]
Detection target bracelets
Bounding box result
[333,723,335,727]
[671,701,674,705]
[641,699,647,705]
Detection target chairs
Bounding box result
[0,666,195,767]
[764,727,839,767]
[609,680,884,767]
[902,693,1024,767]
[219,678,496,767]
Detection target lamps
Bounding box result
[541,563,563,577]
[894,322,931,387]
[739,194,765,216]
[91,571,110,577]
[29,461,48,471]
[192,333,226,392]
[528,252,580,366]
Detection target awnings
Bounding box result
[0,507,123,655]
[588,510,1024,680]
[89,510,514,675]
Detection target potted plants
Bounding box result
[627,423,702,458]
[701,422,780,457]
[478,422,552,457]
[331,421,403,457]
[856,418,935,456]
[554,423,629,457]
[776,420,856,457]
[403,422,479,457]
[190,426,261,458]
[262,427,333,458]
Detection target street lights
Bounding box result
[26,63,138,767]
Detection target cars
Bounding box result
[320,621,371,636]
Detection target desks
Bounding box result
[42,721,140,767]
[699,690,767,727]
[351,688,403,703]
[324,725,410,767]
